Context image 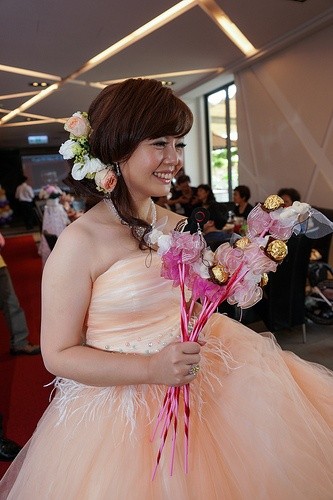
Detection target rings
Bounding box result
[189,365,200,375]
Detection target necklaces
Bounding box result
[104,198,156,244]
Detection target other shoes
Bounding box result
[10,341,41,355]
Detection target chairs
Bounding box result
[240,204,333,344]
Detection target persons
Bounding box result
[151,196,190,205]
[266,188,308,325]
[0,76,333,499]
[15,176,35,227]
[202,203,232,252]
[0,228,40,356]
[197,185,217,208]
[233,184,253,221]
[0,431,21,462]
[176,176,199,206]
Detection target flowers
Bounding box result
[58,111,118,194]
